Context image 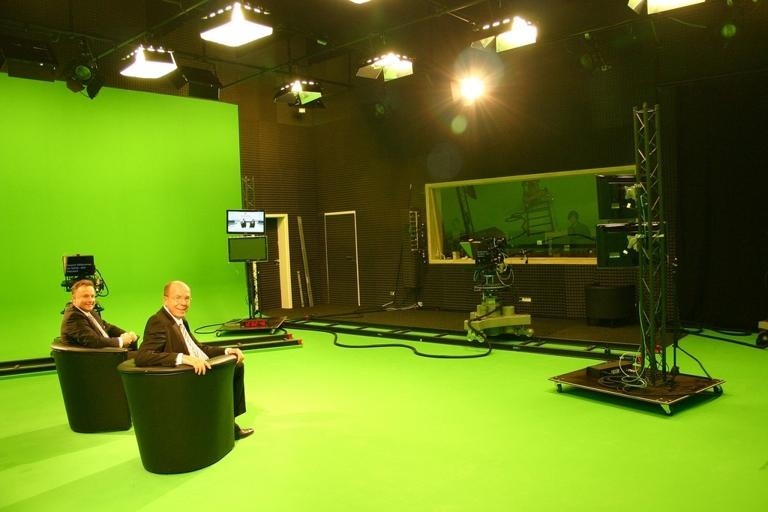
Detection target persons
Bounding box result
[568,210,591,253]
[241,218,246,228]
[134,280,253,441]
[447,218,465,257]
[250,219,255,228]
[61,280,140,360]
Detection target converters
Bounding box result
[586,360,632,379]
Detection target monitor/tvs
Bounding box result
[596,175,638,220]
[596,225,639,268]
[62,256,94,276]
[229,237,268,262]
[459,239,483,259]
[226,210,266,234]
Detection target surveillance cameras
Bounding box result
[66,39,101,99]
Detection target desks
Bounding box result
[506,233,597,256]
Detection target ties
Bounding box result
[89,315,100,333]
[179,324,202,360]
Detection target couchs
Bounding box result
[51,341,138,432]
[586,283,635,328]
[117,355,237,474]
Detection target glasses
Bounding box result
[167,295,192,301]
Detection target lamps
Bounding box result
[470,15,539,53]
[294,108,307,120]
[627,0,704,15]
[585,30,612,78]
[355,51,416,82]
[5,24,58,70]
[373,103,386,119]
[169,62,223,101]
[199,0,277,48]
[64,33,106,99]
[273,80,321,105]
[117,32,177,79]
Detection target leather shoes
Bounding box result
[234,429,254,440]
